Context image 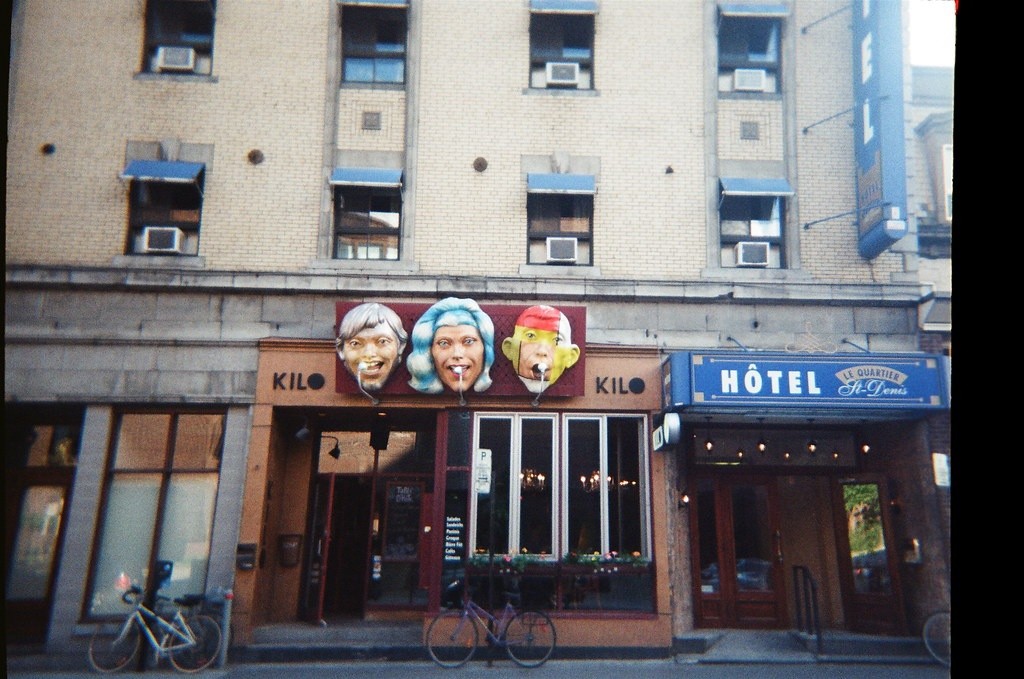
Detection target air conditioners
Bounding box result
[143,226,184,252]
[733,241,770,267]
[545,236,578,262]
[545,62,579,86]
[157,47,195,73]
[734,68,766,91]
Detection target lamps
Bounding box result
[453,365,467,407]
[860,419,871,453]
[321,435,341,459]
[807,419,817,452]
[756,418,768,452]
[704,416,715,451]
[356,362,381,406]
[832,432,838,459]
[532,363,547,407]
[295,418,310,440]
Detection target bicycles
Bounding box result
[920,611,951,669]
[86,585,223,675]
[424,578,557,669]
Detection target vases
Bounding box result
[467,562,643,573]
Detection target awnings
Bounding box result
[336,0,790,35]
[119,159,796,210]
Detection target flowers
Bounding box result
[470,549,644,564]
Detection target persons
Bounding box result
[406,297,496,406]
[335,302,408,404]
[502,305,580,394]
